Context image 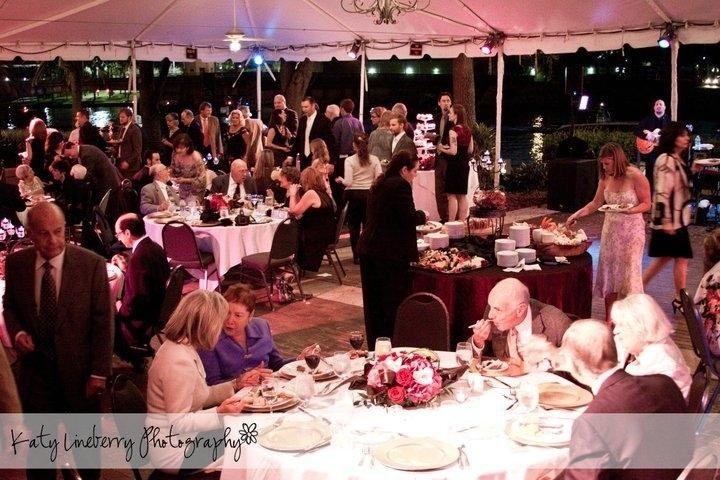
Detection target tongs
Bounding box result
[558,220,577,235]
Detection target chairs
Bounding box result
[107,370,184,478]
[671,287,720,480]
[692,170,719,227]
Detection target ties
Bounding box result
[39,262,57,330]
[166,186,172,197]
[304,117,311,157]
[507,329,520,360]
[233,182,241,200]
[203,118,210,146]
[116,128,127,158]
[437,115,445,156]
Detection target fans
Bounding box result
[201,1,278,53]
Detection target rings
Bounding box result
[97,392,102,395]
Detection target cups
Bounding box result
[542,233,554,243]
[454,342,473,367]
[335,350,353,374]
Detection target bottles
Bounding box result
[179,198,186,215]
[694,134,701,151]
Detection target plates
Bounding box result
[541,383,592,408]
[482,359,531,376]
[147,198,288,227]
[256,420,331,453]
[415,217,465,250]
[503,414,576,447]
[375,438,461,473]
[597,203,634,213]
[239,386,299,413]
[492,225,536,265]
[280,359,338,380]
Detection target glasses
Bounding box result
[115,231,124,238]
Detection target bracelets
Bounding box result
[230,377,240,395]
[661,217,671,223]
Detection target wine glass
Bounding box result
[188,193,274,219]
[519,380,539,436]
[305,345,321,392]
[333,329,392,397]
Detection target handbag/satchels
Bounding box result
[96,207,113,242]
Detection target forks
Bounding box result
[254,417,284,438]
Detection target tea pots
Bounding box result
[0,218,24,242]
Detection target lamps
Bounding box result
[340,0,431,26]
[478,32,506,54]
[654,22,680,48]
[346,39,363,59]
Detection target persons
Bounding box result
[110,209,174,374]
[633,99,670,215]
[693,261,720,360]
[1,201,118,480]
[141,290,262,479]
[0,90,477,287]
[355,150,431,353]
[566,142,653,322]
[701,228,720,274]
[195,281,321,389]
[641,122,696,317]
[561,316,696,480]
[609,293,694,404]
[462,277,573,379]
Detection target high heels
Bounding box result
[672,299,684,314]
